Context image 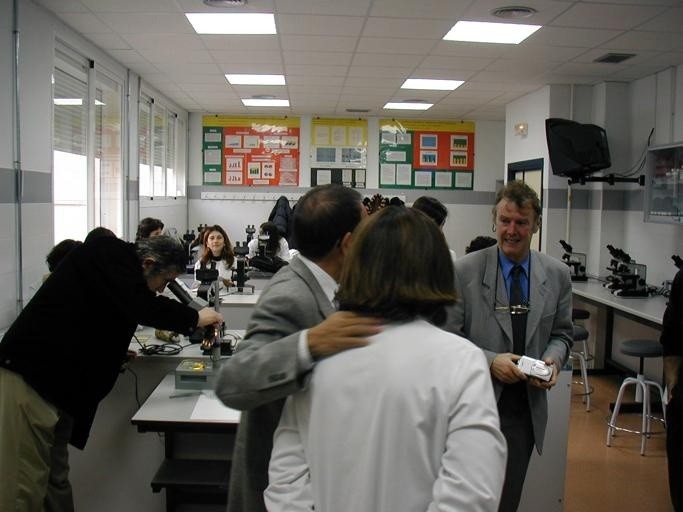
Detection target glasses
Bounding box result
[493,293,531,315]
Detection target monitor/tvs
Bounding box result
[545,118,612,179]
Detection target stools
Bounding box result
[572,307,593,385]
[570,323,596,413]
[608,339,666,457]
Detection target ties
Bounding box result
[508,265,528,335]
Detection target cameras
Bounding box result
[516,356,554,382]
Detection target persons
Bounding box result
[364,195,498,259]
[445,184,572,512]
[247,223,289,260]
[660,266,683,512]
[137,218,165,239]
[263,206,508,512]
[194,225,237,281]
[0,235,223,511]
[216,184,385,512]
[46,227,116,272]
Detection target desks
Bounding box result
[122,246,278,511]
[570,276,670,414]
